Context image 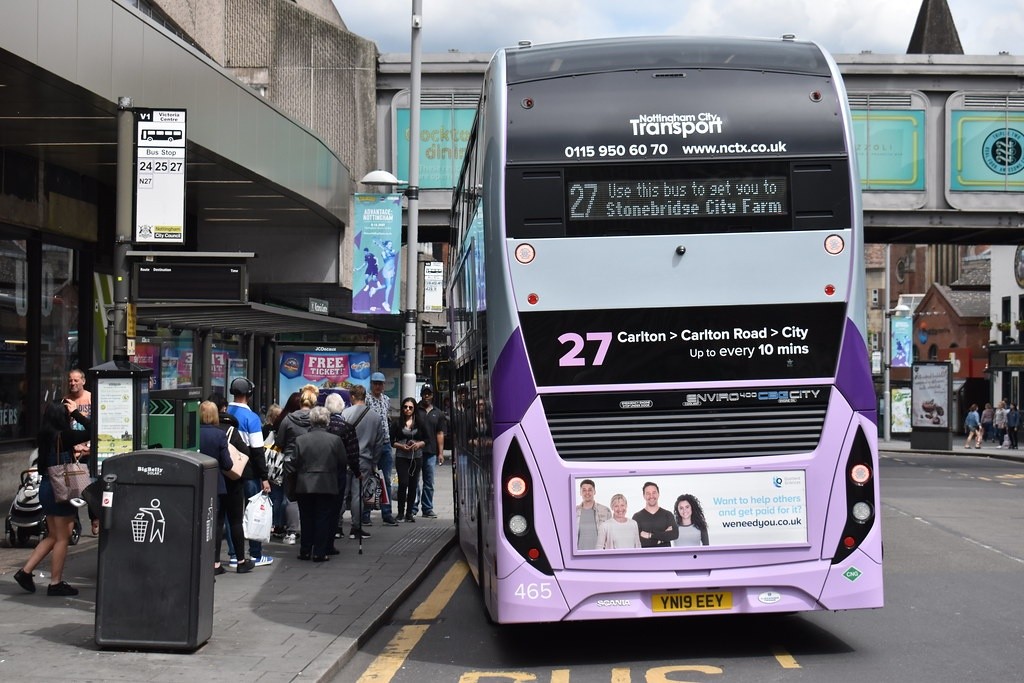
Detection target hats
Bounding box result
[371,372,385,381]
[421,383,434,393]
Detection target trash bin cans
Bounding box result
[95,447,219,649]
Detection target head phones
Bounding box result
[230,378,253,398]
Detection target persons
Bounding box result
[633,482,679,547]
[262,372,398,555]
[390,397,432,523]
[573,480,612,549]
[1008,402,1020,449]
[596,494,642,551]
[964,404,982,449]
[665,495,709,549]
[199,377,274,575]
[357,240,397,312]
[1002,399,1010,410]
[981,404,995,443]
[992,401,1007,448]
[284,406,347,561]
[61,367,99,535]
[14,398,92,595]
[451,382,493,521]
[412,383,445,518]
[896,335,911,366]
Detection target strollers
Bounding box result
[4,446,83,548]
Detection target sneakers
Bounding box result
[383,517,398,526]
[335,527,344,538]
[283,534,296,544]
[236,558,255,572]
[215,565,226,574]
[13,568,37,592]
[229,559,238,568]
[362,519,373,525]
[250,554,273,567]
[349,528,372,538]
[47,580,79,596]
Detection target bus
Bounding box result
[443,35,884,622]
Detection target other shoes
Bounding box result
[312,555,330,562]
[412,513,416,519]
[422,509,437,518]
[997,446,1002,448]
[405,513,416,522]
[298,553,310,560]
[395,513,405,522]
[91,519,99,535]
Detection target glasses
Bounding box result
[403,405,413,410]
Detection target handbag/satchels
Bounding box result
[221,425,249,480]
[243,489,274,544]
[49,430,91,503]
[363,469,390,511]
[264,442,284,486]
